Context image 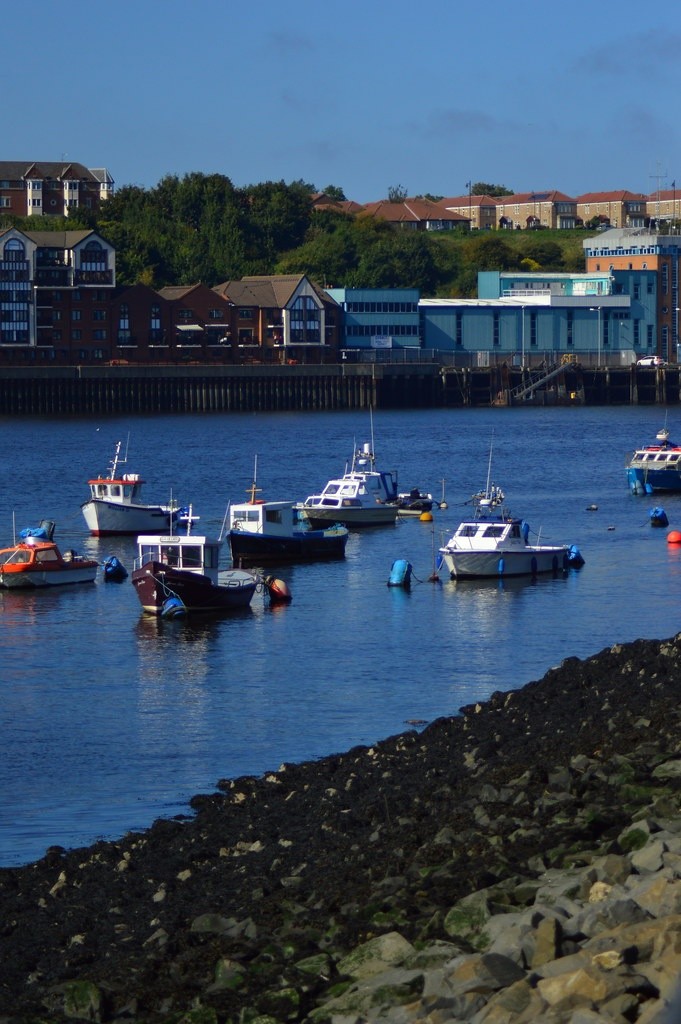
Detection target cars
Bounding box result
[637,355,665,365]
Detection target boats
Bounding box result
[624,409,681,490]
[385,493,432,515]
[292,404,400,527]
[471,428,505,503]
[80,430,183,536]
[438,499,569,580]
[131,488,259,615]
[0,510,99,589]
[224,454,349,567]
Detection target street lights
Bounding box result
[590,308,601,366]
